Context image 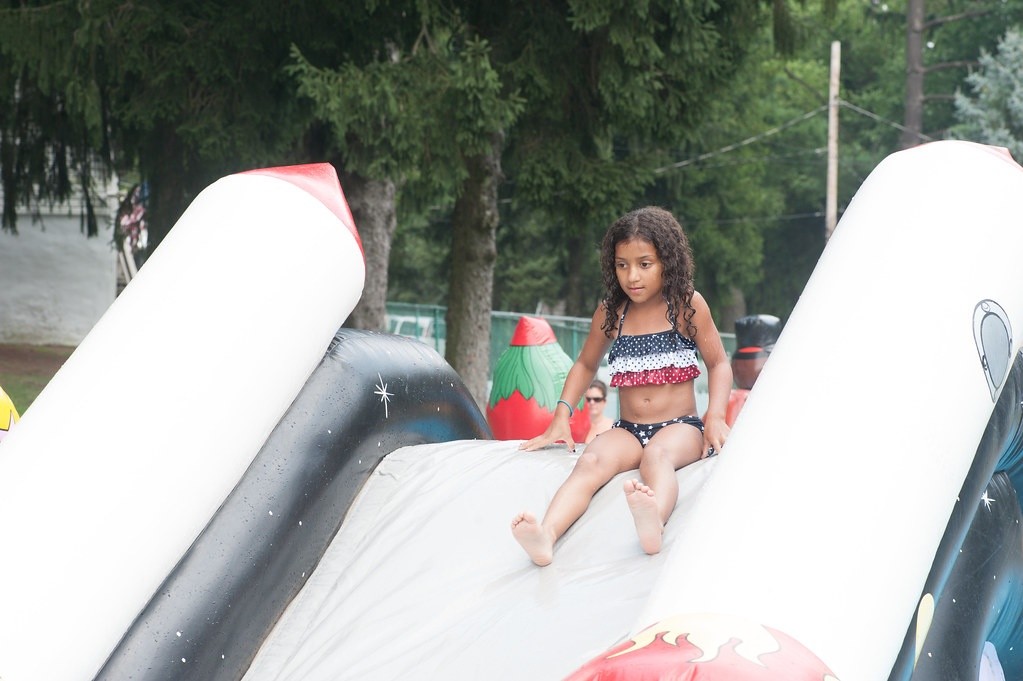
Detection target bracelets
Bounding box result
[557,399,574,418]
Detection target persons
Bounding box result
[579,378,617,442]
[506,203,734,568]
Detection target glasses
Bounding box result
[586,397,604,403]
